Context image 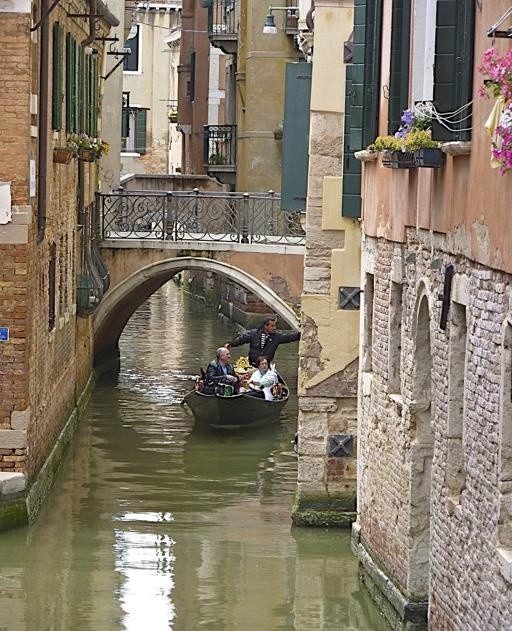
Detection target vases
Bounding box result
[381,149,447,168]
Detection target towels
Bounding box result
[484,96,507,170]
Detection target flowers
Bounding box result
[478,48,512,176]
[69,132,111,162]
[373,102,446,152]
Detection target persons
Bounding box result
[223,316,301,369]
[200,346,239,396]
[242,354,279,401]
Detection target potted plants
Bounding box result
[53,146,73,164]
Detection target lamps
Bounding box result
[264,5,300,34]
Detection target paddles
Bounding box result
[180,332,241,406]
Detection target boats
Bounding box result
[179,362,290,429]
